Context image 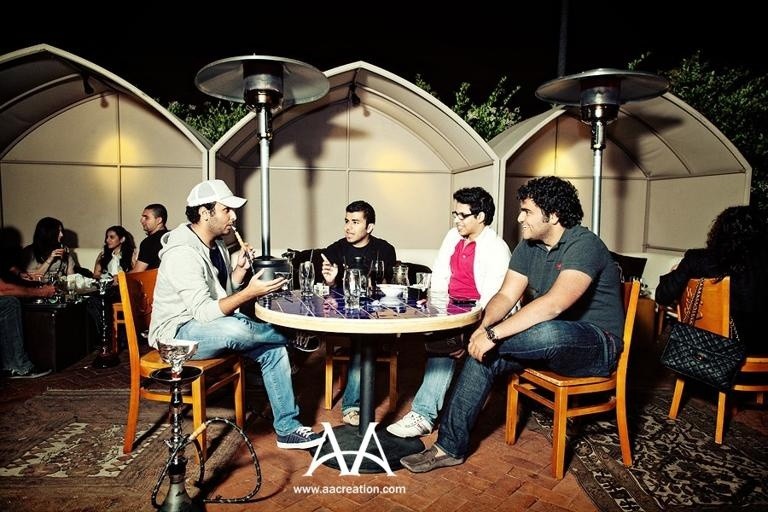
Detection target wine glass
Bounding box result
[157,339,201,376]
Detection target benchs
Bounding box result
[618,248,685,340]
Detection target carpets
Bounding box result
[524,384,768,511]
[0,387,254,511]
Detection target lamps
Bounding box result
[80,71,95,95]
[348,82,361,106]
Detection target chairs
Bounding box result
[668,276,767,448]
[116,265,245,469]
[324,333,404,414]
[111,301,126,352]
[504,275,643,478]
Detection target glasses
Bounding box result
[452,210,476,221]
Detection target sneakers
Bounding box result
[288,329,321,353]
[341,411,361,427]
[422,331,465,356]
[385,411,435,441]
[274,426,327,452]
[399,444,465,475]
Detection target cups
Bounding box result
[298,258,433,311]
[26,270,107,304]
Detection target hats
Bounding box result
[185,178,248,210]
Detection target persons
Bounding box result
[148,179,326,450]
[314,200,396,429]
[0,261,57,379]
[654,206,768,359]
[18,217,76,279]
[400,176,625,472]
[94,226,137,279]
[86,204,169,355]
[386,186,519,439]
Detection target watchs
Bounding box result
[485,328,499,343]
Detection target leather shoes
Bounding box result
[6,365,53,380]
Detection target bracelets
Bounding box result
[45,261,51,266]
[324,281,335,288]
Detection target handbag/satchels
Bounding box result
[655,274,749,395]
